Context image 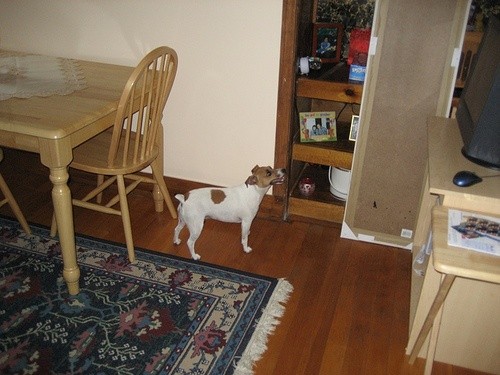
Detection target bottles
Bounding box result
[299,177,316,196]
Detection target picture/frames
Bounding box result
[299,112,337,143]
[311,22,343,62]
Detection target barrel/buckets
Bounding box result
[328,165,352,201]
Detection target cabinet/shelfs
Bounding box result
[288,62,363,223]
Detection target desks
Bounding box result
[405,117,499,375]
[0,49,167,295]
[408,206,500,375]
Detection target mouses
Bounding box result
[453,171,483,187]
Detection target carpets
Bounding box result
[0,214,294,375]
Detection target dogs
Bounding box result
[172,164,288,260]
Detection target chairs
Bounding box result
[50,46,179,262]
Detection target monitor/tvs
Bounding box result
[455,14,500,170]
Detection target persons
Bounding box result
[310,124,328,136]
[316,38,330,54]
